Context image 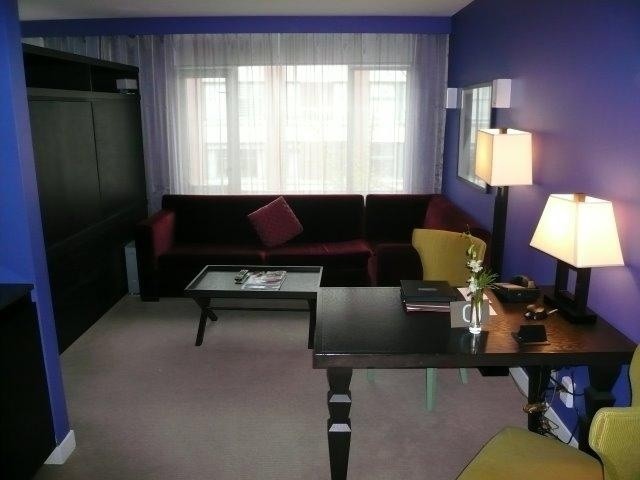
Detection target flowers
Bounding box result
[465,246,500,327]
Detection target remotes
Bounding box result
[235,275,249,284]
[235,269,249,281]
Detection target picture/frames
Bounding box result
[456,80,496,194]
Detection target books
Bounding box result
[241,270,287,291]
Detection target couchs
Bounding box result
[135,194,490,302]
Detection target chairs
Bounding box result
[455,345,640,480]
[365,229,487,412]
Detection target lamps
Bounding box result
[475,127,533,283]
[530,192,625,326]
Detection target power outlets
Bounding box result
[559,374,576,408]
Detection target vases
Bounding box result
[468,296,483,334]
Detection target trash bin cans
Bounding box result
[124,240,140,293]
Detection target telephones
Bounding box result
[492,275,543,303]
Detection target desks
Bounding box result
[312,286,638,480]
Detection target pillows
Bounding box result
[248,197,303,247]
